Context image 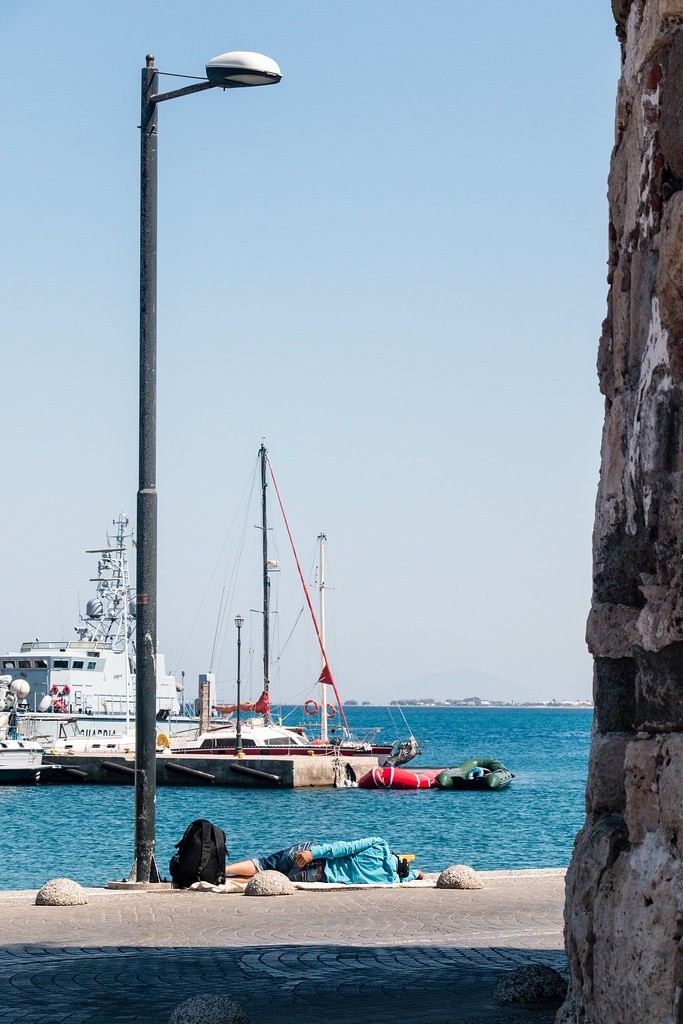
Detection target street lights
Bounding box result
[107,50,285,891]
[233,614,246,758]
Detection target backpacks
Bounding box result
[169,819,230,888]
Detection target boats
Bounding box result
[358,767,447,790]
[0,431,424,787]
[437,757,513,790]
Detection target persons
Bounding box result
[225,836,424,884]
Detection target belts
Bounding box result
[316,863,322,880]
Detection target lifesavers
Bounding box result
[158,734,169,747]
[304,699,318,714]
[326,705,336,717]
